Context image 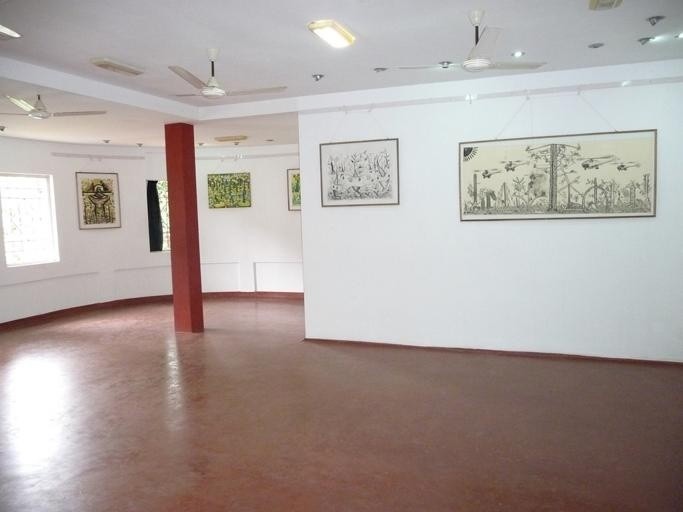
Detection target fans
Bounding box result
[168,47,290,99]
[0,92,106,119]
[391,9,548,73]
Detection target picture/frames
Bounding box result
[205,172,253,210]
[458,129,658,223]
[320,137,399,208]
[287,168,301,212]
[75,172,121,229]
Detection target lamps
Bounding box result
[215,136,249,141]
[0,24,22,41]
[90,58,143,78]
[589,0,625,11]
[308,19,356,50]
